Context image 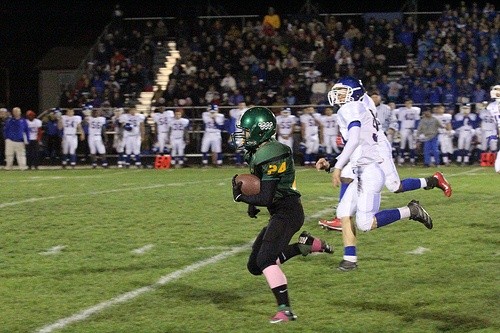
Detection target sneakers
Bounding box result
[298,231,335,254]
[334,260,358,272]
[318,218,342,232]
[270,310,296,321]
[432,171,451,198]
[407,199,433,229]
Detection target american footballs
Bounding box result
[235,174,260,196]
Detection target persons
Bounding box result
[415,20,500,63]
[110,104,190,168]
[262,7,281,30]
[215,5,228,16]
[400,58,500,115]
[369,92,498,168]
[486,85,500,174]
[232,106,334,324]
[111,2,125,21]
[437,0,500,23]
[407,0,417,19]
[60,21,168,116]
[228,101,344,169]
[0,107,108,170]
[394,14,417,54]
[295,16,402,116]
[319,95,452,232]
[316,76,433,272]
[201,103,225,169]
[150,19,301,115]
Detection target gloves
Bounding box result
[247,204,260,219]
[232,174,243,202]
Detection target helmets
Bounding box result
[241,106,277,150]
[124,123,133,131]
[81,104,93,115]
[47,108,59,122]
[333,76,366,107]
[207,104,218,112]
[445,103,456,114]
[280,107,291,116]
[460,103,470,113]
[299,142,306,152]
